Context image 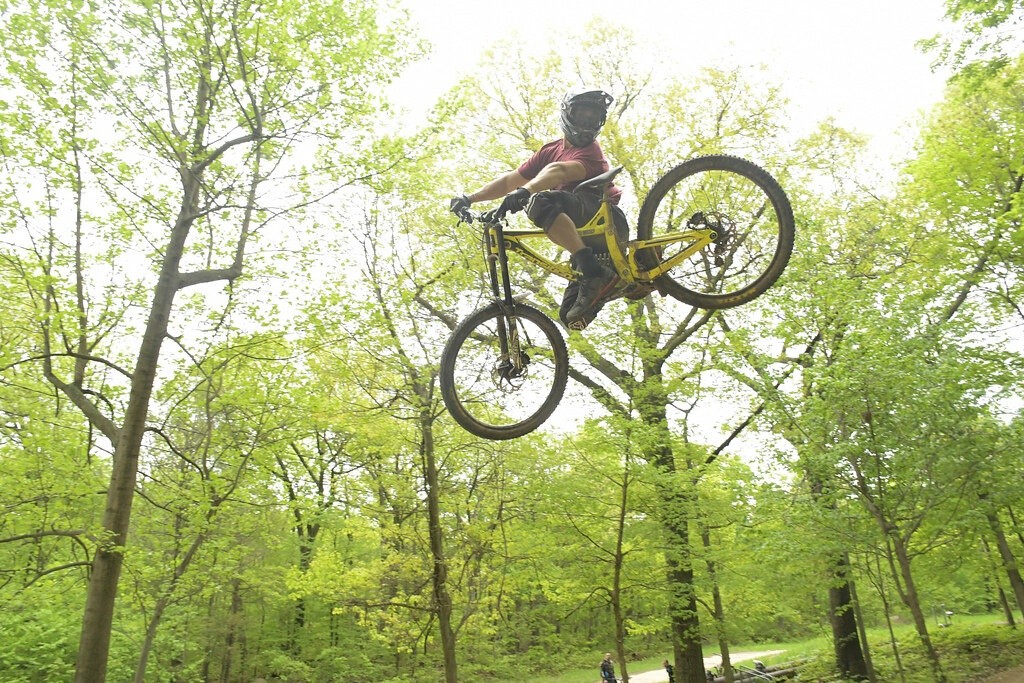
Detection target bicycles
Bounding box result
[438,155,796,441]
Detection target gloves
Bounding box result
[450,194,471,215]
[495,187,530,217]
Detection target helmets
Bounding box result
[560,91,614,147]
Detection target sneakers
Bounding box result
[566,266,619,321]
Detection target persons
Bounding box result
[663,659,674,683]
[599,653,617,683]
[450,88,662,330]
